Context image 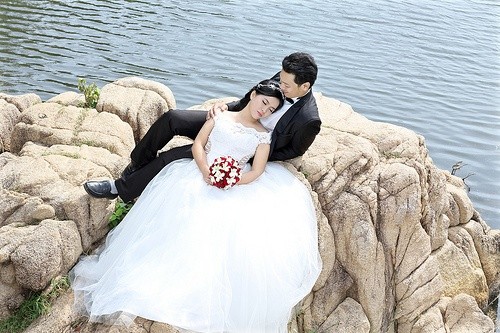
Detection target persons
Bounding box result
[160,80,286,288]
[83,51,322,203]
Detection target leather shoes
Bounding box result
[121,163,137,176]
[83,180,119,199]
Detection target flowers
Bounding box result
[209,155,245,191]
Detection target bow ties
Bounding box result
[284,95,294,103]
